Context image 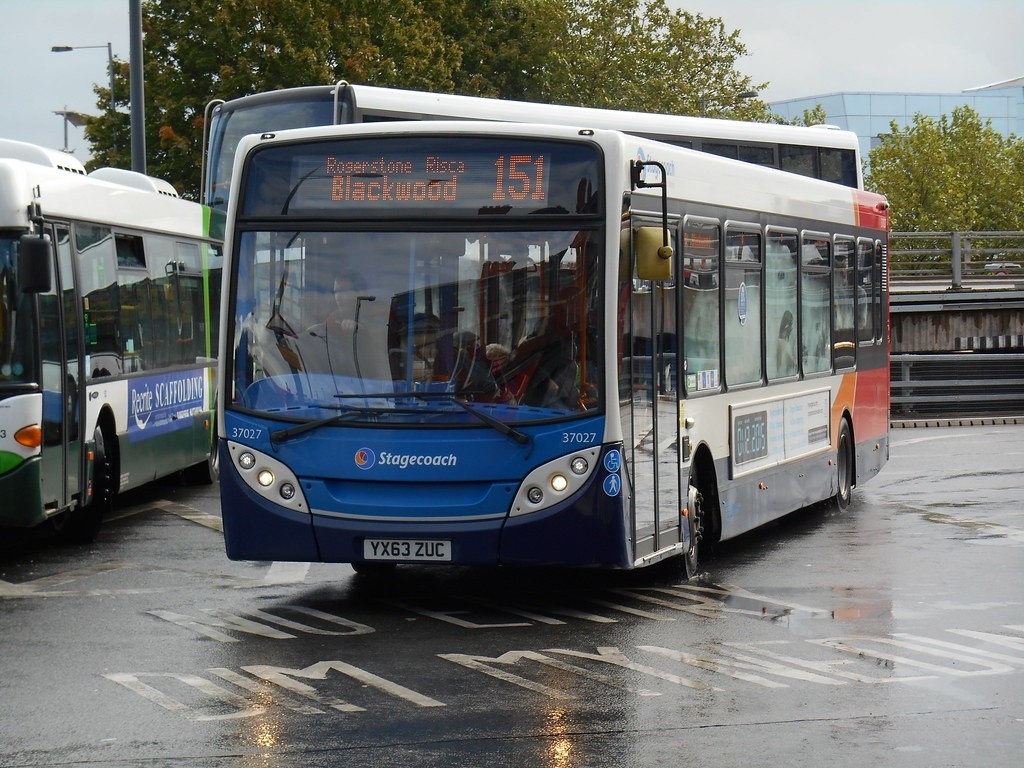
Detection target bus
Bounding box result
[214,120,891,581]
[199,80,864,215]
[0,138,227,547]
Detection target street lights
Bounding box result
[52,42,118,154]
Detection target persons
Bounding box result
[320,271,390,375]
[452,313,593,409]
